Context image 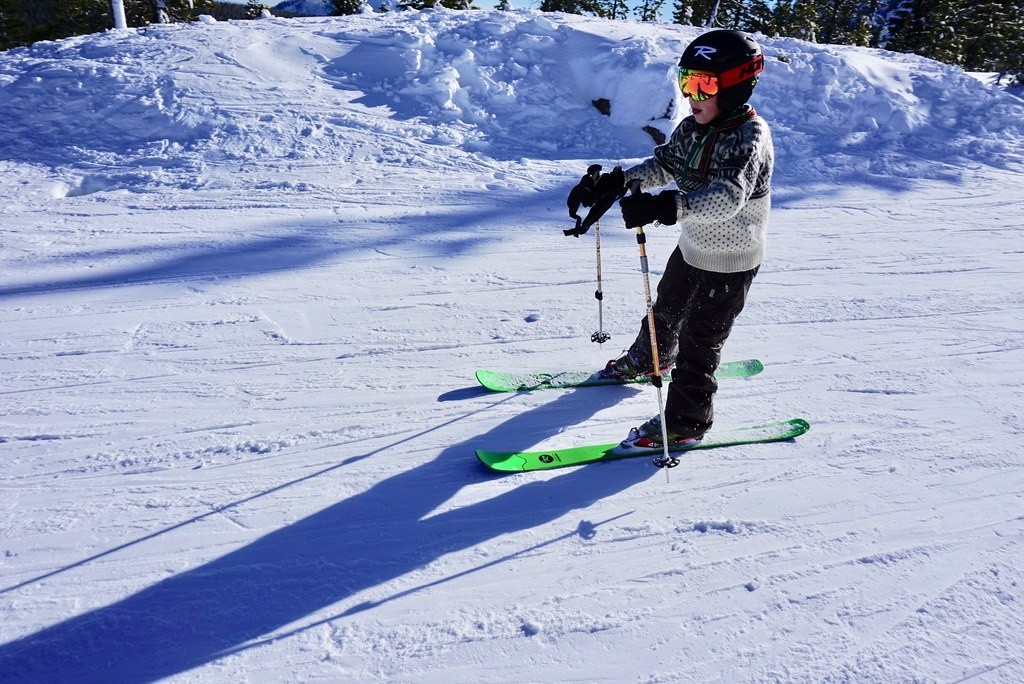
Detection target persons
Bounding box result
[567,31,777,446]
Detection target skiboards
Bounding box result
[472,356,812,472]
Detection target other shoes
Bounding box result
[601,354,679,382]
[613,412,706,454]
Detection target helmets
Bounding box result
[679,30,764,110]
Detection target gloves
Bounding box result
[582,167,624,208]
[620,191,676,228]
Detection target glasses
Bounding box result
[678,69,718,99]
